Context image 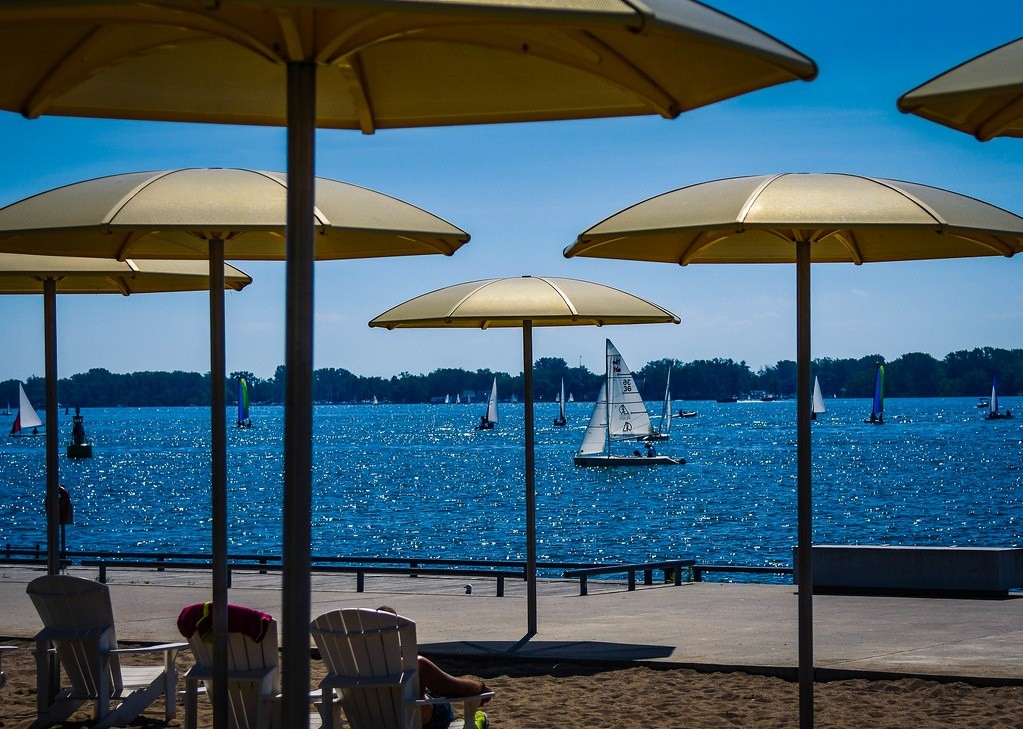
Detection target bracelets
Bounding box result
[478,680,487,693]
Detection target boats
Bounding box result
[670,412,696,417]
[977,401,989,407]
[715,397,737,403]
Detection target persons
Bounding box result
[32,427,38,434]
[367,604,496,728]
[645,443,657,457]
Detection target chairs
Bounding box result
[25,574,191,729]
[176,601,340,729]
[309,608,496,729]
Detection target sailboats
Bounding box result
[984,376,1011,419]
[0,401,12,416]
[231,377,255,429]
[467,395,471,404]
[8,381,46,436]
[456,394,460,404]
[554,377,575,425]
[810,375,826,420]
[373,395,378,406]
[864,365,886,424]
[444,393,450,405]
[475,377,499,430]
[574,341,680,466]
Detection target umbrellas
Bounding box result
[1,166,470,729]
[0,250,253,628]
[899,33,1022,143]
[1,1,821,729]
[367,275,683,635]
[564,171,1023,729]
